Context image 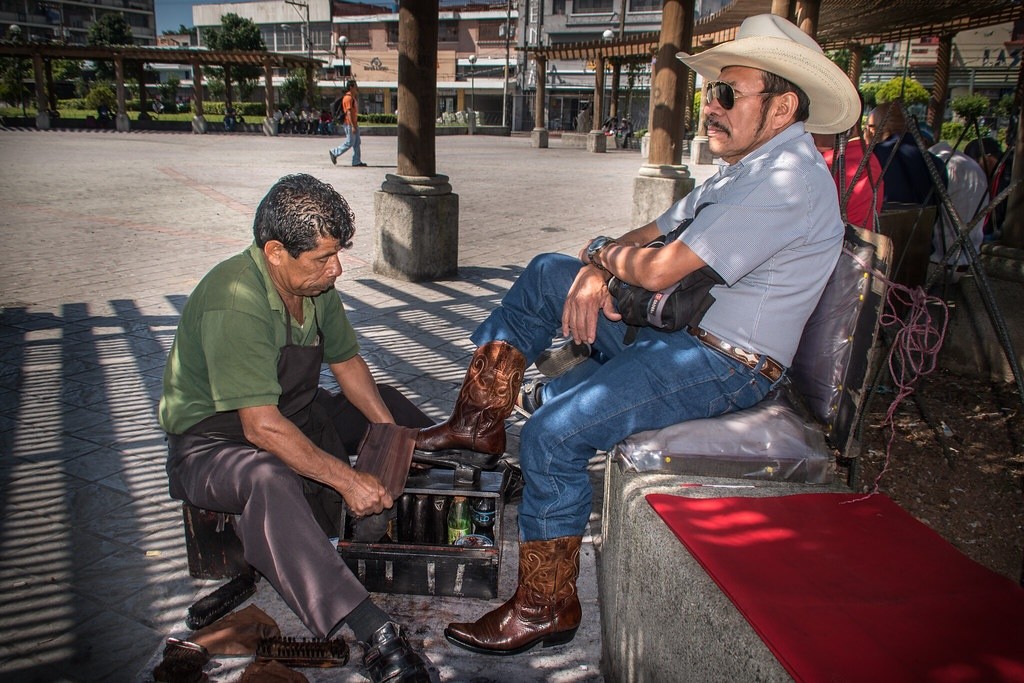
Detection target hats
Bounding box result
[675,12,861,134]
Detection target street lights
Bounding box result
[337,35,349,95]
[280,24,312,135]
[9,24,26,116]
[468,54,477,135]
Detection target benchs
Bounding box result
[562,134,617,149]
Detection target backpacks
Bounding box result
[607,202,723,346]
[329,96,354,124]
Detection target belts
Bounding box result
[686,326,784,383]
[929,261,969,272]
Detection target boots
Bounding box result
[443,515,584,656]
[411,340,529,470]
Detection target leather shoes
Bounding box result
[493,459,524,503]
[357,619,431,683]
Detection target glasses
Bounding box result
[705,79,784,110]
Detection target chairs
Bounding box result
[610,218,892,491]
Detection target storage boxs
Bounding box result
[335,467,507,599]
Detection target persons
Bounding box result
[284,105,299,134]
[823,122,883,232]
[602,116,618,136]
[328,112,335,124]
[620,117,634,149]
[272,105,282,133]
[864,100,949,258]
[223,100,237,133]
[175,95,191,113]
[411,14,862,655]
[158,171,524,683]
[96,98,111,129]
[914,121,989,370]
[963,136,1015,239]
[318,108,332,134]
[153,99,164,114]
[237,114,246,124]
[330,81,367,167]
[310,108,320,134]
[576,106,589,134]
[298,107,309,135]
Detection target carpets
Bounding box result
[644,493,1024,683]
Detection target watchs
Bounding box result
[588,236,619,271]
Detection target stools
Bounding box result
[183,502,261,583]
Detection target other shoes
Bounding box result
[329,150,337,165]
[352,162,367,166]
